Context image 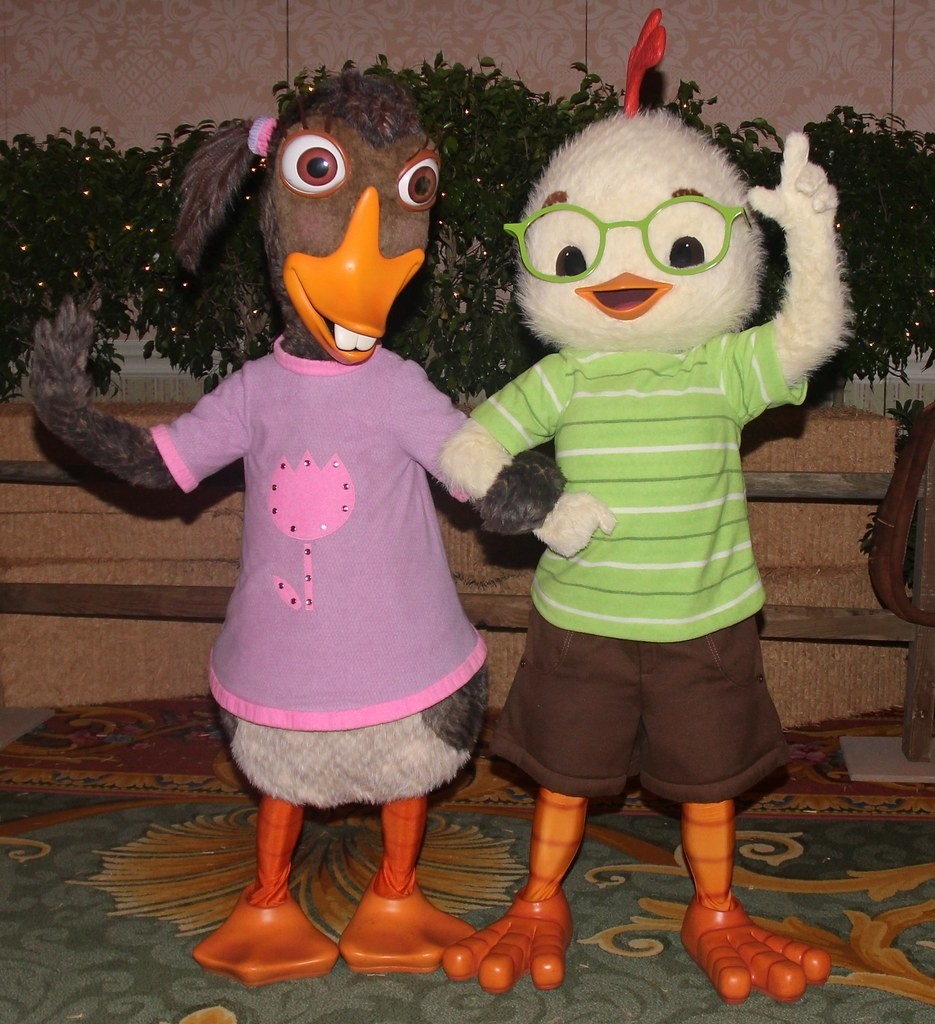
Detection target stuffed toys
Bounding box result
[30,62,606,985]
[439,99,864,1014]
